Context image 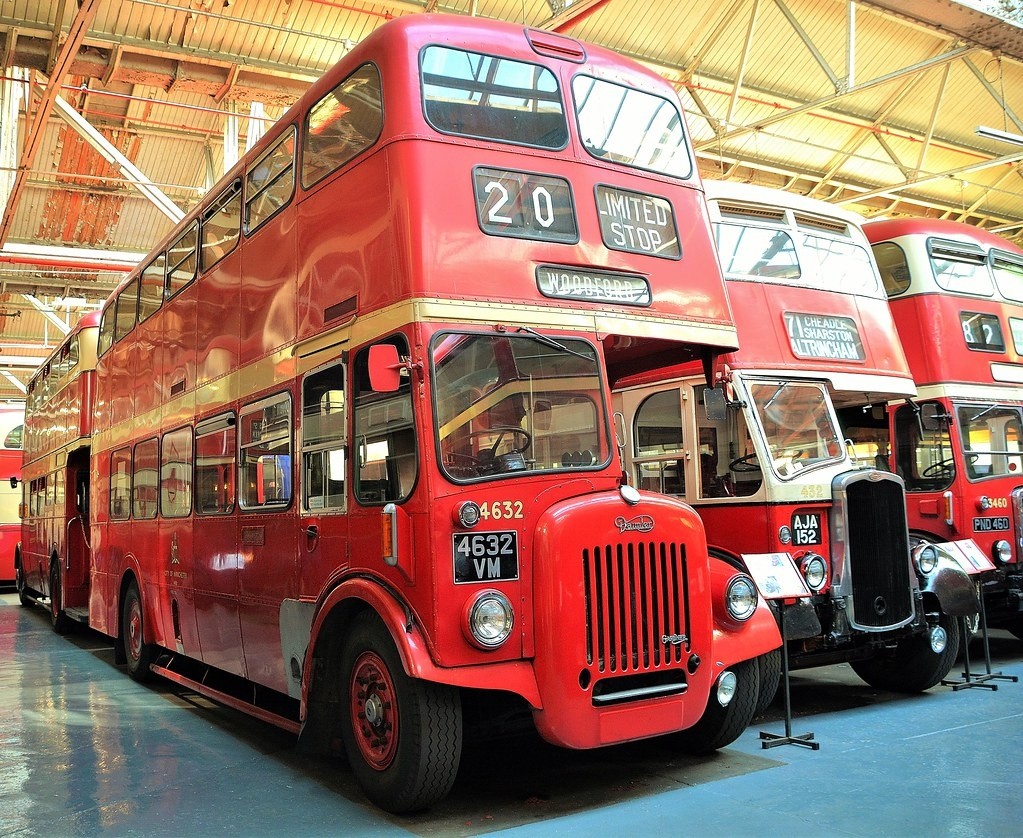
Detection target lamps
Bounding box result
[973,127,1023,147]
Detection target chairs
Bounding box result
[392,432,418,499]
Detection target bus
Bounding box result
[86,10,785,819]
[10,309,106,636]
[833,217,1023,645]
[610,178,982,720]
